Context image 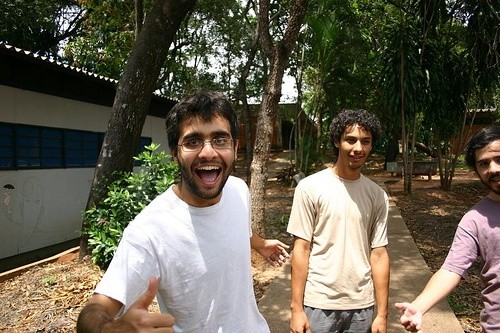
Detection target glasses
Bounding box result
[176,136,235,152]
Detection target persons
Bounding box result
[77,91,290,333]
[395,124,500,333]
[287,109,389,333]
